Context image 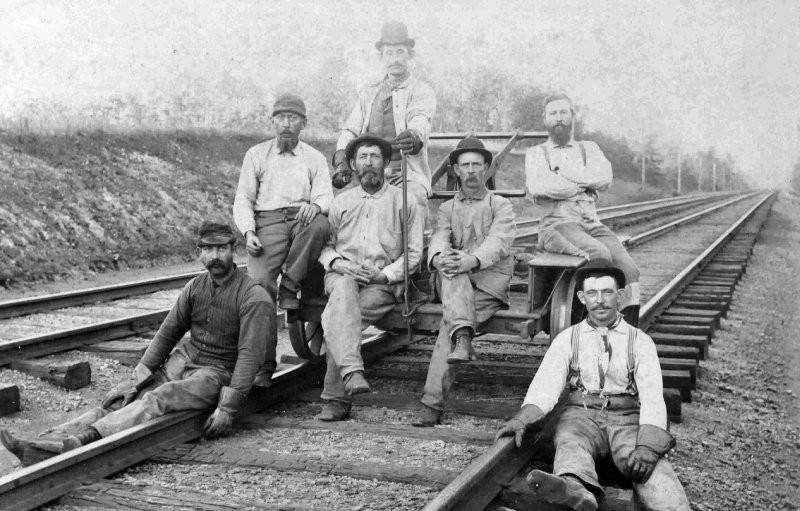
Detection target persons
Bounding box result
[408,137,519,429]
[230,92,336,389]
[492,258,693,511]
[0,220,276,470]
[522,92,642,330]
[328,21,439,264]
[316,132,424,424]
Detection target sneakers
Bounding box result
[343,372,375,396]
[253,359,278,391]
[527,467,603,511]
[276,284,301,310]
[319,398,352,421]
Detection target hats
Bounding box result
[376,21,412,49]
[273,92,306,120]
[572,257,627,291]
[344,134,392,163]
[197,219,234,247]
[449,135,491,165]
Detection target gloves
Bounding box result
[101,360,153,409]
[495,403,545,447]
[623,424,678,485]
[203,385,249,442]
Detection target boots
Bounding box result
[414,403,443,427]
[1,424,101,467]
[446,331,477,365]
